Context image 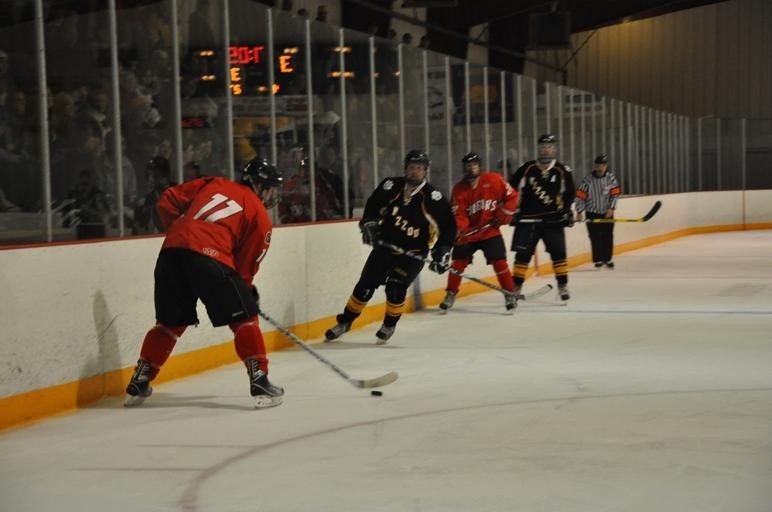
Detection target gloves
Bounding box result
[360,221,382,246]
[428,252,453,275]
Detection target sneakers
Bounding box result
[606,262,615,267]
[595,262,603,267]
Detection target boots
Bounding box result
[126,359,160,397]
[375,324,395,341]
[557,284,570,301]
[324,321,351,340]
[439,288,456,311]
[244,358,286,397]
[501,288,518,310]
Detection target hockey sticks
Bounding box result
[520,201,661,224]
[379,240,554,301]
[257,310,398,389]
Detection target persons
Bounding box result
[507,134,576,303]
[575,154,621,268]
[0,0,430,236]
[439,152,519,312]
[125,159,284,399]
[324,148,457,340]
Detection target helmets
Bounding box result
[594,154,609,165]
[537,134,560,164]
[239,155,286,210]
[461,152,482,177]
[403,148,430,183]
